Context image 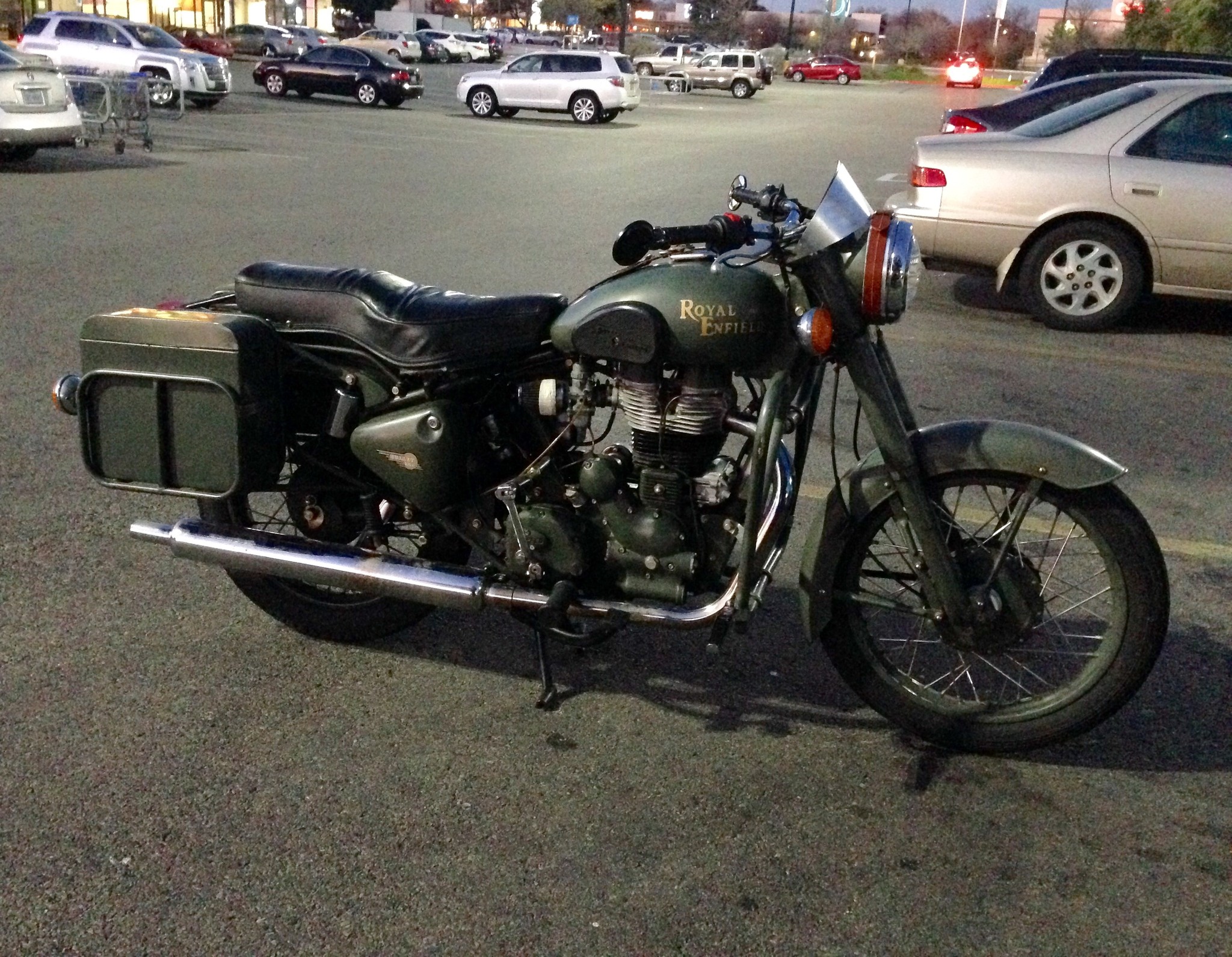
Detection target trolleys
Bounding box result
[61,68,154,155]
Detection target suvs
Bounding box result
[17,10,232,109]
[341,29,422,61]
[457,49,642,125]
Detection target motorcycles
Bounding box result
[52,160,1172,765]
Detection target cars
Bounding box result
[415,35,445,65]
[252,44,425,108]
[783,55,862,85]
[939,71,1232,135]
[663,51,774,99]
[168,27,235,57]
[946,59,983,90]
[414,23,581,63]
[883,77,1232,335]
[280,25,341,50]
[0,40,87,158]
[1022,49,1232,92]
[215,23,306,58]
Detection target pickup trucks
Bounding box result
[632,45,718,76]
